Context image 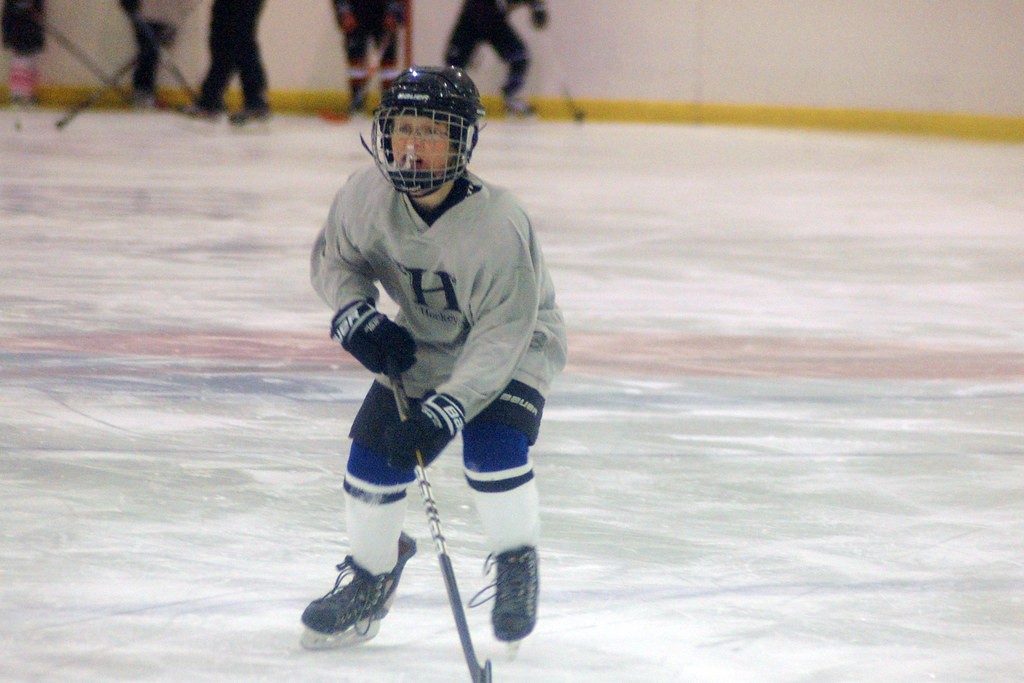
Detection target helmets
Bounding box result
[371,63,488,198]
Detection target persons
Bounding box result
[332,0,413,118]
[183,0,272,133]
[297,66,570,660]
[444,0,549,116]
[2,0,50,106]
[118,0,177,115]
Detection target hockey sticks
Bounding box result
[40,18,136,106]
[366,296,493,683]
[53,50,141,130]
[128,11,199,109]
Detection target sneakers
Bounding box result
[503,92,539,120]
[125,87,171,112]
[347,87,368,122]
[299,530,417,652]
[176,90,226,131]
[227,95,271,137]
[491,546,540,660]
[5,55,38,114]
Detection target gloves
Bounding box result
[382,387,466,472]
[340,13,357,34]
[382,14,398,34]
[329,299,420,378]
[533,10,546,29]
